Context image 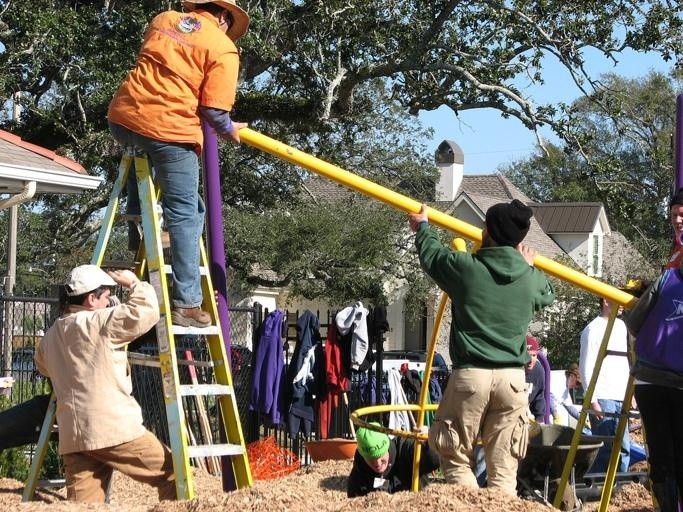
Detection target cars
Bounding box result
[382,351,449,374]
[231,344,253,386]
[0,349,35,371]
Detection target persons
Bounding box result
[622,265,682,512]
[470,335,544,493]
[30,264,176,509]
[406,197,554,494]
[579,294,636,480]
[341,421,439,496]
[659,188,683,270]
[106,2,249,330]
[549,362,588,431]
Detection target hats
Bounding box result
[182,0,250,42]
[486,199,532,247]
[669,187,683,209]
[356,422,390,459]
[527,335,540,351]
[64,264,118,297]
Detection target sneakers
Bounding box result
[171,306,212,328]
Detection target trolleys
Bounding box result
[516,424,604,512]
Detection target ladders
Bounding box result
[21,142,255,503]
